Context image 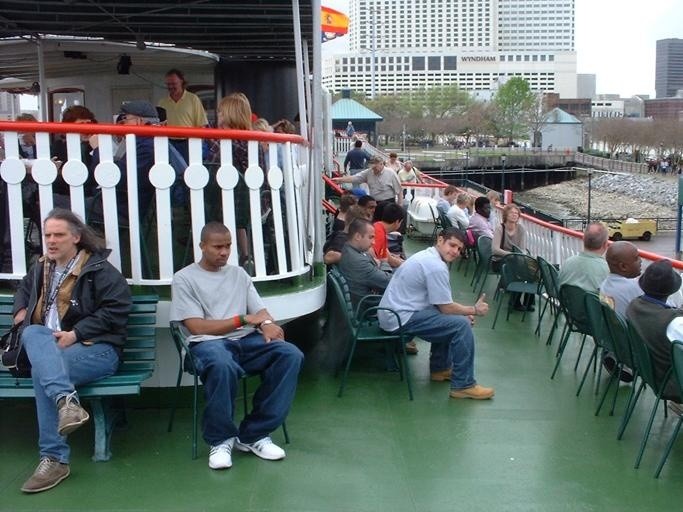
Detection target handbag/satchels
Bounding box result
[492,222,506,271]
[3,321,31,380]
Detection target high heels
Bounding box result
[239,254,249,266]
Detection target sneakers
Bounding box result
[20,455,70,493]
[667,400,683,419]
[234,435,287,461]
[401,344,419,355]
[208,435,237,471]
[56,401,91,437]
[449,382,495,400]
[430,368,452,382]
[603,350,633,382]
[409,341,417,346]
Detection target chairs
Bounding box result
[534,253,577,336]
[166,319,293,460]
[330,267,418,401]
[596,300,636,417]
[491,253,545,339]
[615,317,669,440]
[633,341,683,480]
[0,294,159,462]
[437,205,469,271]
[427,201,445,245]
[455,218,480,278]
[550,283,602,396]
[471,235,509,304]
[584,291,652,393]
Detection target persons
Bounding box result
[447,193,469,230]
[558,223,610,298]
[333,193,357,232]
[350,135,359,151]
[338,217,419,354]
[372,202,406,267]
[324,204,373,265]
[437,186,457,215]
[89,100,188,276]
[330,155,403,208]
[346,121,356,137]
[469,197,494,240]
[12,208,133,494]
[168,221,304,470]
[252,119,285,193]
[397,161,425,203]
[14,112,43,258]
[492,204,536,312]
[377,228,495,400]
[293,113,308,135]
[156,69,209,166]
[179,93,271,271]
[358,195,377,217]
[111,114,127,161]
[344,140,371,189]
[616,151,619,160]
[50,106,97,222]
[273,120,297,134]
[374,200,396,223]
[599,241,676,383]
[625,260,683,404]
[647,157,678,177]
[383,152,401,174]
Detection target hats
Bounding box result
[120,99,159,118]
[368,155,383,163]
[637,258,682,298]
[401,160,413,167]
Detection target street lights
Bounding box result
[500,153,507,204]
[465,146,469,189]
[585,164,595,225]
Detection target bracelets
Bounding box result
[234,315,241,327]
[239,314,247,326]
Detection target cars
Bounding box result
[598,216,657,241]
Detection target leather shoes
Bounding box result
[527,304,535,312]
[515,302,526,312]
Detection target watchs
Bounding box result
[260,319,274,328]
